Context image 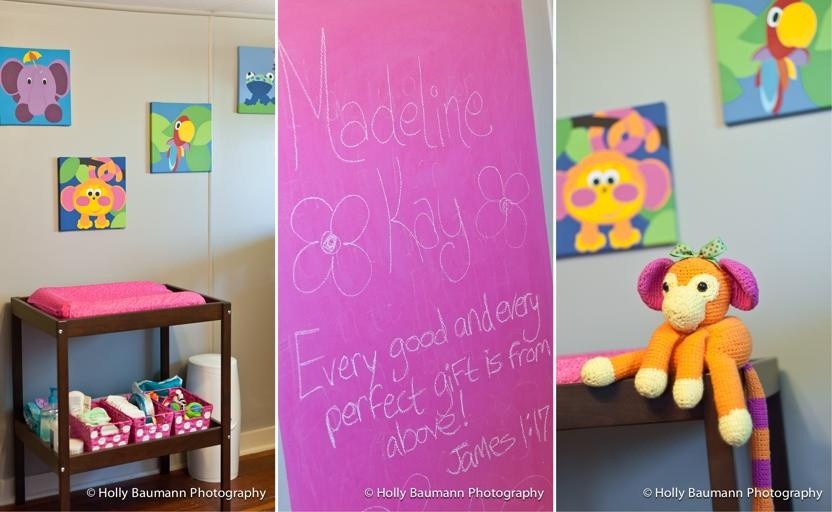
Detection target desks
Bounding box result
[11,285,231,512]
[556,352,794,511]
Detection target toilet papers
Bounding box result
[186,354,240,483]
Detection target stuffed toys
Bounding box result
[578,235,776,512]
